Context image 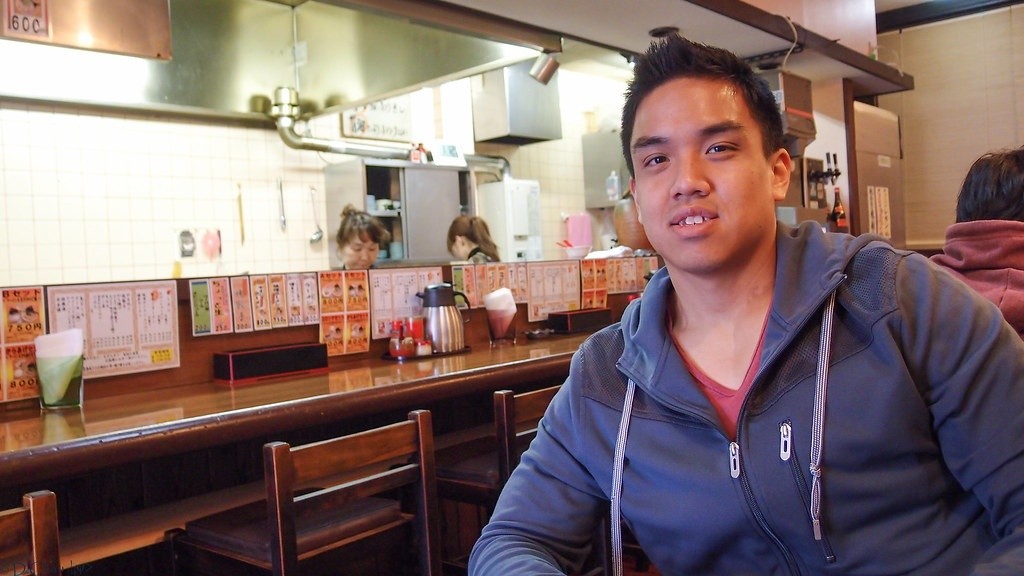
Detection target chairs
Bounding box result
[390,384,608,576]
[0,490,63,576]
[166,409,443,576]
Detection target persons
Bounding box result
[336,206,392,270]
[448,216,499,262]
[467,33,1024,576]
[927,148,1023,340]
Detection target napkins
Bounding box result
[483,287,517,338]
[34,328,84,405]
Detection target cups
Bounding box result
[484,308,518,348]
[33,354,86,411]
[389,242,403,260]
[367,194,376,212]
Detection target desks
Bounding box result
[0,325,593,531]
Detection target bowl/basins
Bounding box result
[561,246,591,259]
[376,198,401,213]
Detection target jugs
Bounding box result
[416,280,472,353]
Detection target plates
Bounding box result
[521,328,555,340]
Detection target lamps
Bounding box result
[529,52,560,85]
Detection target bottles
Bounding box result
[606,170,622,201]
[387,316,432,357]
[830,187,849,235]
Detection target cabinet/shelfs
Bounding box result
[324,161,478,268]
